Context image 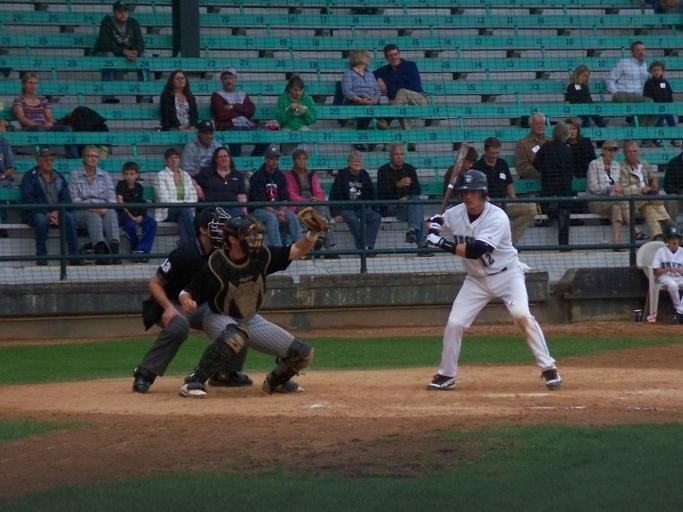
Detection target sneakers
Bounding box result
[638,138,683,148]
[671,312,683,324]
[358,243,377,256]
[404,234,417,243]
[427,373,457,391]
[178,379,208,398]
[70,244,149,266]
[300,236,339,259]
[417,248,435,257]
[36,252,47,265]
[407,143,417,152]
[377,120,390,129]
[263,377,305,394]
[541,368,562,390]
[352,143,368,152]
[132,368,155,393]
[368,142,377,152]
[0,230,10,238]
[209,371,253,387]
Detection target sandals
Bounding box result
[635,232,649,240]
[611,240,625,252]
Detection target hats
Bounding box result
[602,139,619,151]
[265,147,280,157]
[113,0,130,12]
[38,148,56,157]
[221,66,237,77]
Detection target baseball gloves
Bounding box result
[297,206,328,233]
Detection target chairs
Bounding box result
[634,240,681,323]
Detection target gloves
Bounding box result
[424,214,445,231]
[424,233,445,248]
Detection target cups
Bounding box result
[633,309,641,324]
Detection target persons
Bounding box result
[425,170,563,390]
[642,0,682,29]
[1,41,430,264]
[175,207,329,399]
[130,207,252,395]
[444,41,683,251]
[91,0,153,104]
[652,224,682,325]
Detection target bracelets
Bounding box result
[440,238,456,254]
[306,229,317,242]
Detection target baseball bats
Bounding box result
[439,143,470,214]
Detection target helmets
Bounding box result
[664,226,683,239]
[224,216,265,254]
[454,169,488,194]
[193,208,231,246]
